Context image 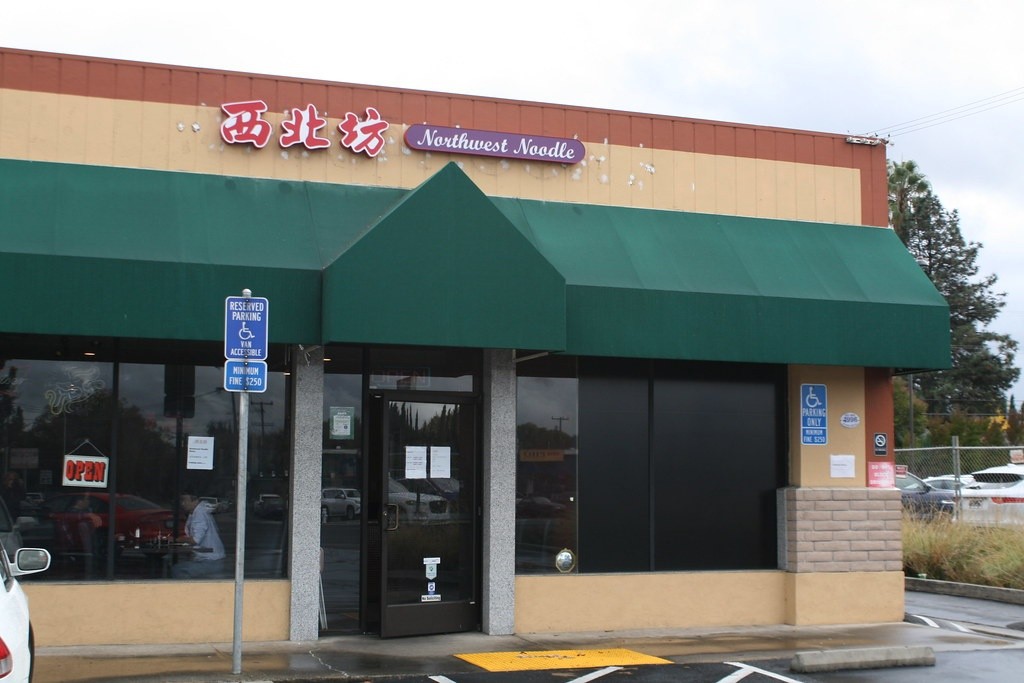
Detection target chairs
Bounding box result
[77,518,101,579]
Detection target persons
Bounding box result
[167,487,226,581]
[1,471,102,581]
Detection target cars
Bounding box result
[199,495,222,514]
[516,495,569,516]
[389,476,451,525]
[903,476,969,501]
[323,488,361,515]
[40,491,191,573]
[955,463,1024,523]
[1,497,39,564]
[0,535,50,682]
[254,494,283,516]
[896,470,957,522]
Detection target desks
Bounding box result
[119,546,214,579]
